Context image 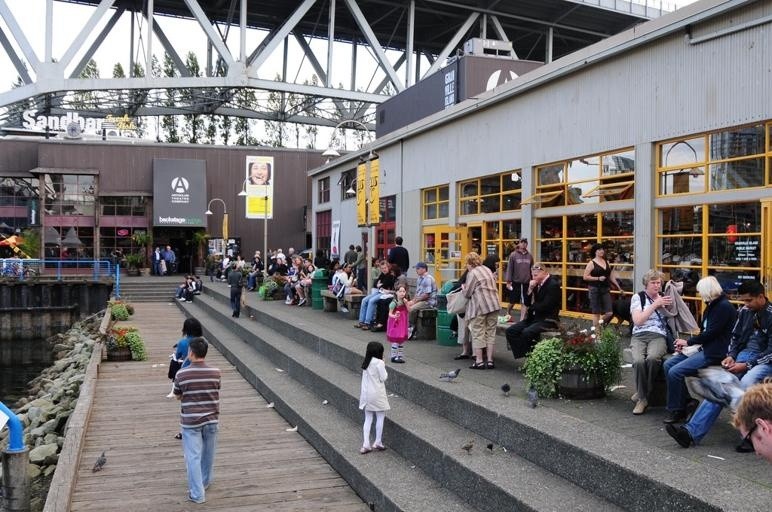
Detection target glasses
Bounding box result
[520,239,527,243]
[531,266,544,272]
[740,425,758,448]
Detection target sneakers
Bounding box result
[632,398,647,414]
[684,399,699,421]
[189,494,204,503]
[736,443,755,452]
[665,422,691,448]
[362,324,370,329]
[471,356,476,363]
[353,322,364,328]
[455,354,467,360]
[631,393,638,402]
[297,297,307,307]
[663,412,683,423]
[408,326,416,340]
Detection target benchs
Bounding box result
[495,321,576,341]
[414,309,438,340]
[622,348,675,407]
[254,277,263,291]
[320,290,366,319]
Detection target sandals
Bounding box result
[469,361,484,369]
[487,361,494,369]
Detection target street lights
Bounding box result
[346,167,369,253]
[237,175,269,292]
[321,120,374,302]
[663,139,706,261]
[204,199,228,261]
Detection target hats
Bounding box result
[412,262,428,268]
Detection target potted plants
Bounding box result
[125,254,140,277]
[132,230,152,277]
[193,232,211,277]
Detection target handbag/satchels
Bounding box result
[370,319,384,332]
[237,279,244,287]
[333,278,345,300]
[681,343,703,358]
[168,359,183,382]
[665,328,675,354]
[446,288,468,314]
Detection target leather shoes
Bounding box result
[360,447,372,453]
[399,356,405,363]
[391,357,401,363]
[373,442,385,450]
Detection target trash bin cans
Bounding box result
[311,269,327,309]
[436,280,461,346]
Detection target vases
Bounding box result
[553,360,606,400]
[107,346,132,362]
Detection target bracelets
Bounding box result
[746,361,748,372]
[597,276,600,282]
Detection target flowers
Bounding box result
[106,326,148,362]
[523,322,622,401]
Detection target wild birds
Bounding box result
[461,439,475,454]
[92,450,108,472]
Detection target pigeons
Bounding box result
[502,384,510,395]
[528,383,539,408]
[440,369,462,383]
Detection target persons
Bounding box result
[630,269,676,415]
[462,252,502,369]
[358,342,391,454]
[175,273,202,303]
[168,317,204,439]
[164,244,177,273]
[372,264,409,332]
[246,247,314,306]
[504,237,535,322]
[173,337,222,504]
[388,236,409,277]
[217,254,246,318]
[665,280,772,453]
[352,259,395,329]
[450,254,500,361]
[728,383,772,463]
[151,247,165,276]
[371,259,381,287]
[314,245,368,299]
[582,243,626,335]
[387,284,418,363]
[661,276,737,424]
[407,261,438,340]
[505,262,562,369]
[248,162,271,185]
